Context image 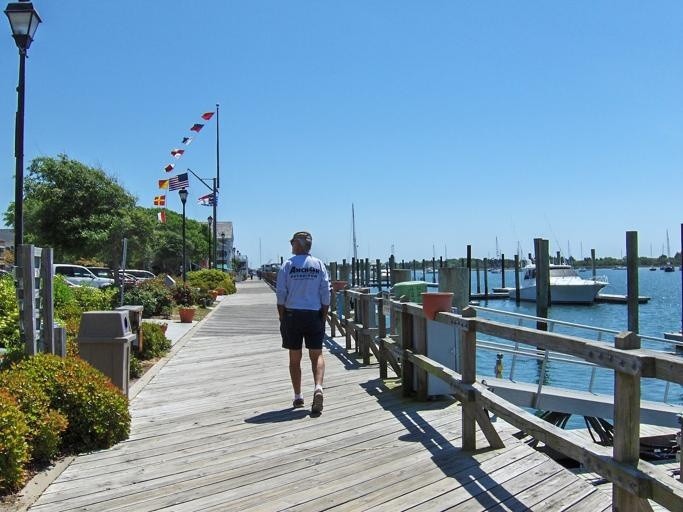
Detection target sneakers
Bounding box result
[294,399,305,408]
[312,389,323,413]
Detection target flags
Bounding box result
[158,179,168,189]
[168,173,188,192]
[198,193,217,206]
[170,149,184,159]
[181,137,194,147]
[163,163,174,173]
[154,196,165,206]
[155,211,166,225]
[190,124,203,133]
[200,112,213,121]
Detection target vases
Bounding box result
[331,279,348,291]
[418,290,454,321]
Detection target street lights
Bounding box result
[178,185,189,281]
[207,216,241,275]
[3,0,42,290]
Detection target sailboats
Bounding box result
[613,248,627,270]
[648,228,683,271]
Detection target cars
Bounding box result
[53,264,176,291]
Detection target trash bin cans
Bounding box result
[393,282,458,400]
[79,309,136,395]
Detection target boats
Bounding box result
[488,235,608,304]
[261,262,281,272]
[380,269,391,281]
[427,267,439,274]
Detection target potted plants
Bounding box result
[208,281,224,300]
[178,303,195,323]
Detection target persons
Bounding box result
[276,231,331,413]
